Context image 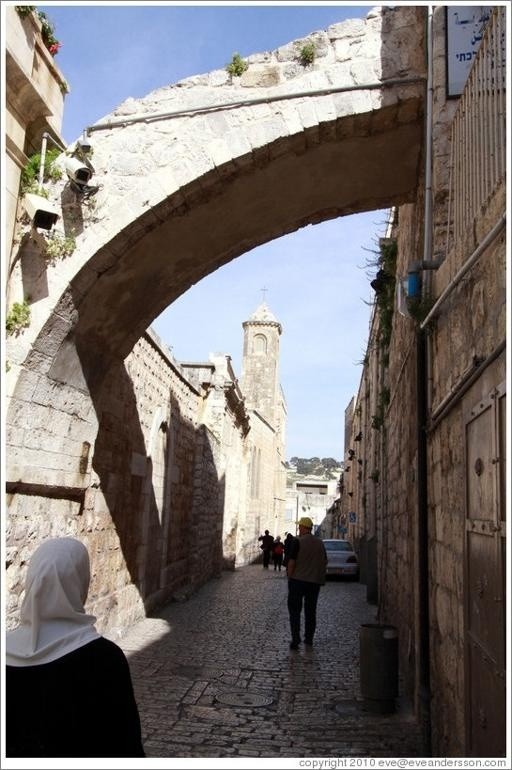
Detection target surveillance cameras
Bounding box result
[66,157,92,184]
[77,140,91,155]
[25,193,61,230]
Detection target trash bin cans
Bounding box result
[358,623,398,701]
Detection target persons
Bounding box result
[287,517,327,646]
[258,529,293,572]
[4,536,147,757]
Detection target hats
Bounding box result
[294,517,313,528]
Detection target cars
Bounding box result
[321,535,358,580]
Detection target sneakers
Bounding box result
[289,638,313,649]
